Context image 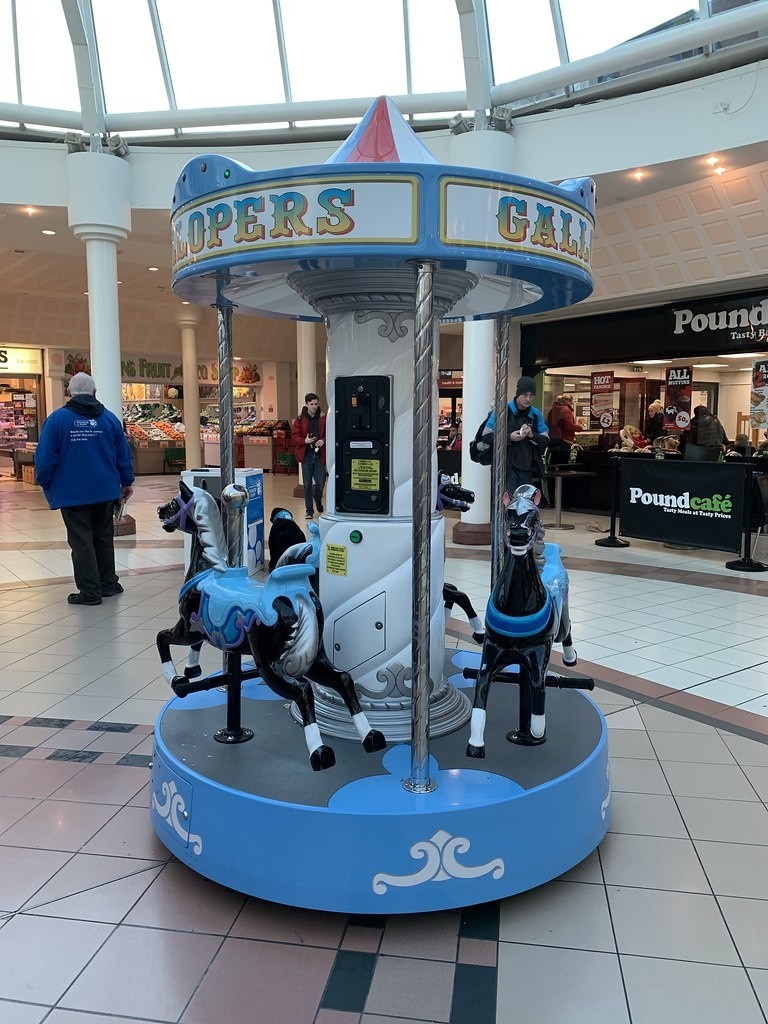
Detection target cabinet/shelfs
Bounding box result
[200,398,256,467]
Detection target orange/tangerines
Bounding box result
[154,422,185,439]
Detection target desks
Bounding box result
[544,471,597,530]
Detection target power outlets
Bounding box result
[712,102,730,114]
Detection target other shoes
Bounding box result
[102,583,124,597]
[67,593,103,606]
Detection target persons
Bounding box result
[630,429,647,448]
[643,399,665,446]
[291,392,326,519]
[483,377,550,537]
[689,405,730,448]
[726,434,758,457]
[758,429,768,455]
[34,373,135,604]
[548,394,584,465]
[438,425,462,451]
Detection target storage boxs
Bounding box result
[236,420,298,474]
[113,514,136,536]
[22,465,38,484]
[126,423,186,448]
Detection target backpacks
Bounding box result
[470,408,493,466]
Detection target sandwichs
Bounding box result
[590,393,613,418]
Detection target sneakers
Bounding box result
[305,509,314,519]
[314,498,323,512]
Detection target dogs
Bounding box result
[268,507,306,570]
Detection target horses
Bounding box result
[156,480,387,770]
[465,484,577,759]
[436,469,485,644]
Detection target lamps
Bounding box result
[105,134,130,158]
[65,132,86,154]
[492,107,515,133]
[448,113,470,135]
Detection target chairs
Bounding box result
[744,476,768,560]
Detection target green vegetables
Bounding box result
[123,402,178,422]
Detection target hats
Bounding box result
[68,371,95,397]
[648,399,661,413]
[516,377,536,397]
